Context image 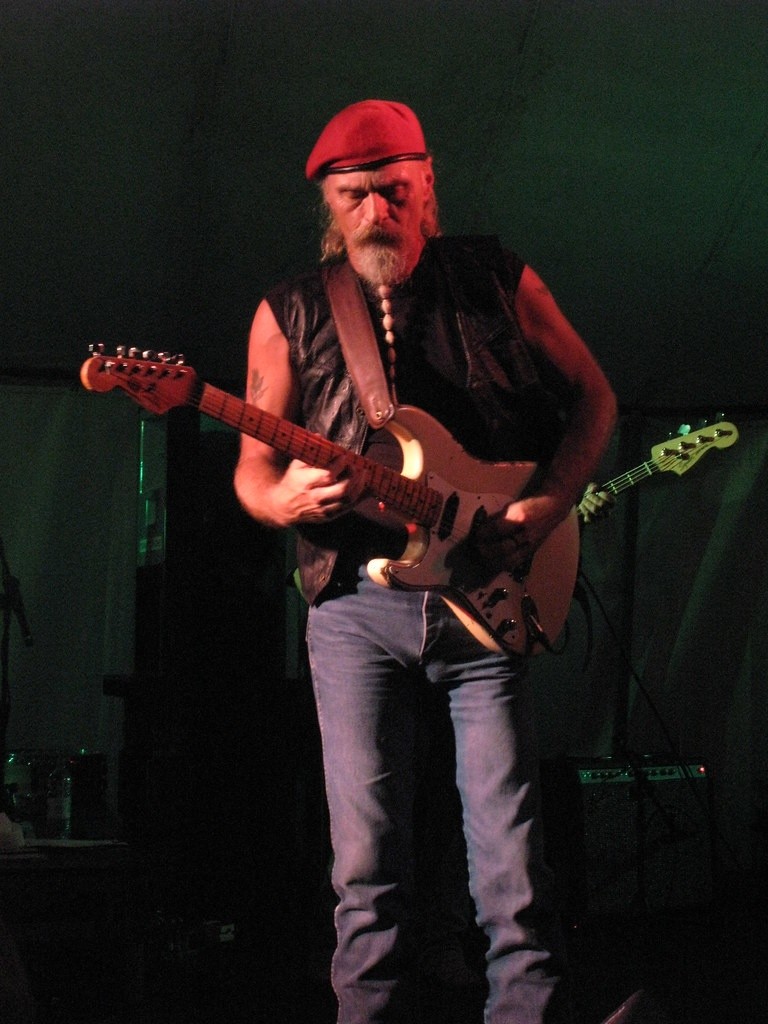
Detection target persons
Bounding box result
[233,97,618,1024]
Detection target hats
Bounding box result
[305,99,430,181]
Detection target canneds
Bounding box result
[1,750,102,841]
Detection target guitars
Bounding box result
[582,419,739,521]
[80,341,579,662]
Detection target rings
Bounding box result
[509,536,521,551]
